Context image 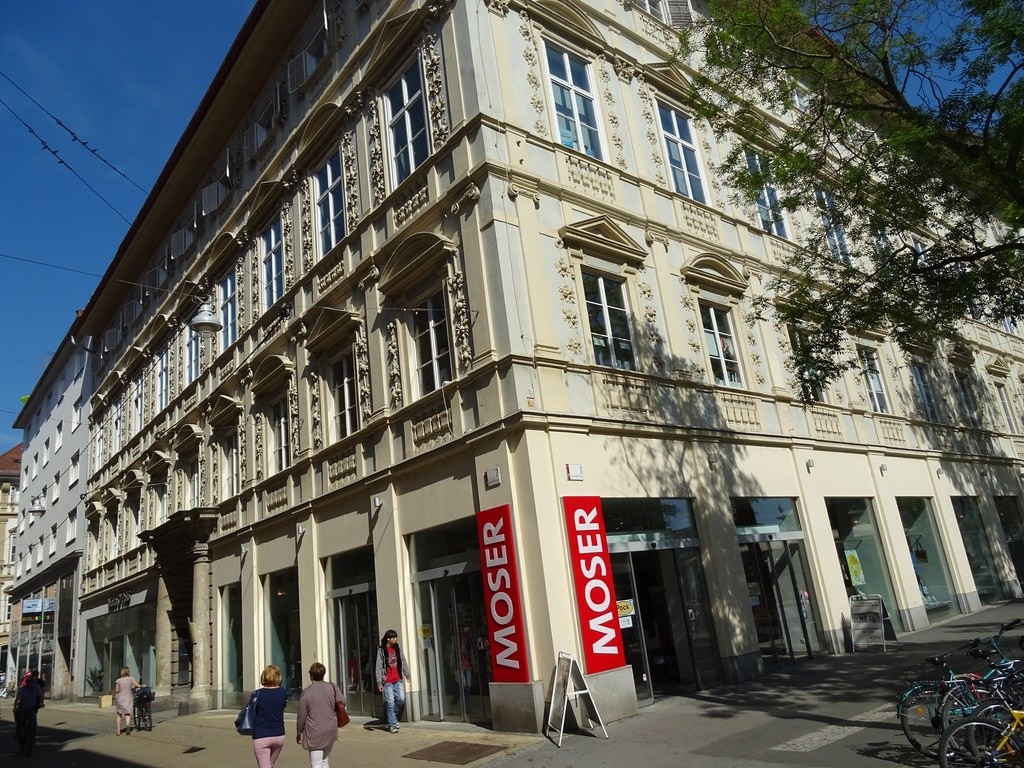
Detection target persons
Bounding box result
[12,677,45,742]
[296,662,348,767]
[375,629,411,734]
[114,667,148,736]
[246,665,288,768]
[29,670,46,705]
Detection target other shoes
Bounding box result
[388,725,397,733]
[395,720,400,728]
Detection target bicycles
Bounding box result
[894,618,1024,768]
[13,702,45,759]
[133,685,154,732]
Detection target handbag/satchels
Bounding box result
[237,690,260,735]
[329,681,350,728]
